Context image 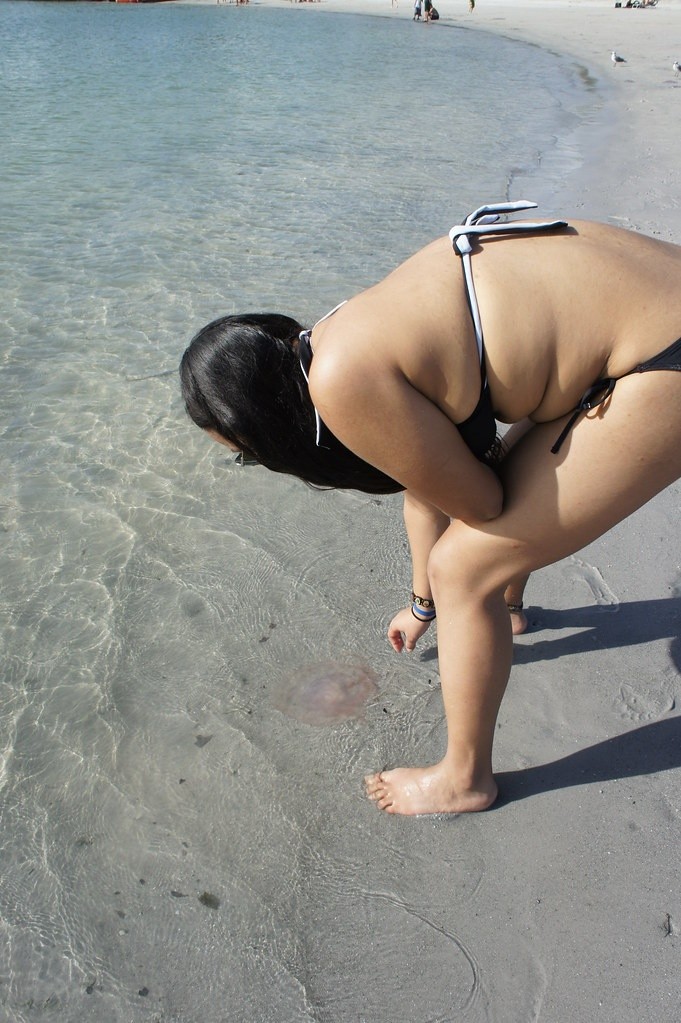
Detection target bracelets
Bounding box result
[414,604,435,616]
[411,593,435,608]
[411,607,436,623]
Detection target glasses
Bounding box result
[234,450,258,467]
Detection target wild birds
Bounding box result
[610,51,627,67]
[672,61,681,78]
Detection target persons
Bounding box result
[179,201,681,816]
[422,0,439,22]
[412,0,423,22]
[626,0,645,8]
[468,0,475,13]
